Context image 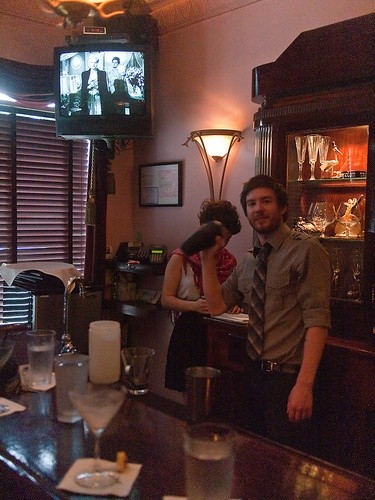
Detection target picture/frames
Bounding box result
[137,162,183,207]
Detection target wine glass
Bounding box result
[307,202,362,239]
[295,133,339,182]
[67,384,126,488]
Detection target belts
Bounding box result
[257,360,299,375]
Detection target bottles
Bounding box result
[89,321,122,384]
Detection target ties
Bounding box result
[243,243,271,369]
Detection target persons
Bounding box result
[198,175,331,456]
[161,199,243,404]
[82,55,125,115]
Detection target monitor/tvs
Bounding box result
[53,44,153,141]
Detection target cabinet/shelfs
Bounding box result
[281,128,368,315]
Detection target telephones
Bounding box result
[148,245,167,263]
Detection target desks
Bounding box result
[203,316,375,459]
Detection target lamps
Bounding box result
[183,129,247,200]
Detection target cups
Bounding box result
[120,347,156,395]
[53,353,89,424]
[186,366,222,425]
[27,329,55,385]
[182,422,236,499]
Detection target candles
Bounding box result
[86,321,121,383]
